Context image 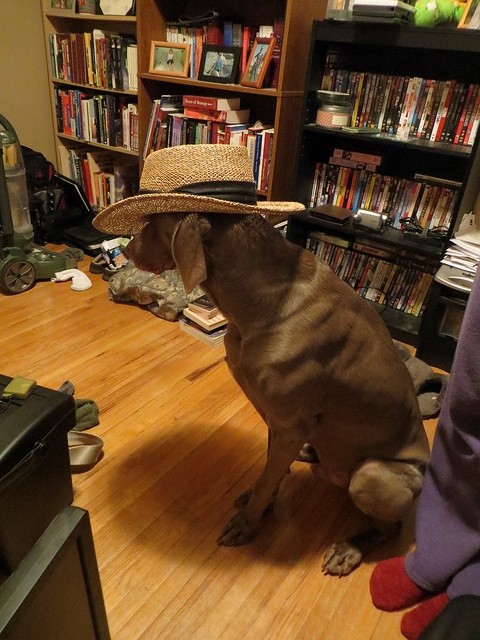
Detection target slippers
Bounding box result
[415,373,450,420]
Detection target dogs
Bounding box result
[124,211,432,579]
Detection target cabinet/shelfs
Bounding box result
[0,506,111,640]
[286,19,479,349]
[137,0,330,202]
[40,0,138,211]
[414,265,476,375]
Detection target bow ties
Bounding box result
[102,264,125,281]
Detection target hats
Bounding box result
[91,144,306,236]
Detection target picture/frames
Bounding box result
[149,40,191,78]
[240,37,277,86]
[198,43,242,84]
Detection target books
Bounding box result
[49,29,137,91]
[54,145,138,211]
[53,88,138,152]
[308,147,464,239]
[314,44,480,148]
[142,93,275,193]
[101,236,129,268]
[178,294,229,346]
[165,8,284,91]
[305,232,435,314]
[47,0,136,15]
[434,212,479,292]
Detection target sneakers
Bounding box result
[90,253,109,273]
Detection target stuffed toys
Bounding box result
[412,0,467,29]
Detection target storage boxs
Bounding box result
[1,374,76,577]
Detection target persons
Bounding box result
[212,52,225,76]
[167,49,176,69]
[249,46,265,82]
[371,262,479,635]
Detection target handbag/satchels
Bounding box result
[108,260,207,322]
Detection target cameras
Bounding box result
[352,210,389,232]
